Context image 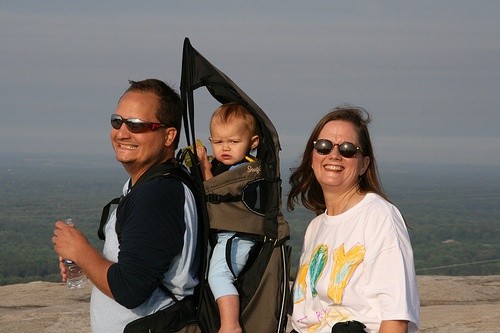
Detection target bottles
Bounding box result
[62,218,89,289]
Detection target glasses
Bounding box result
[111,114,166,133]
[314,139,359,158]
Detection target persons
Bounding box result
[52,79,207,333]
[196,103,260,333]
[287,104,420,333]
